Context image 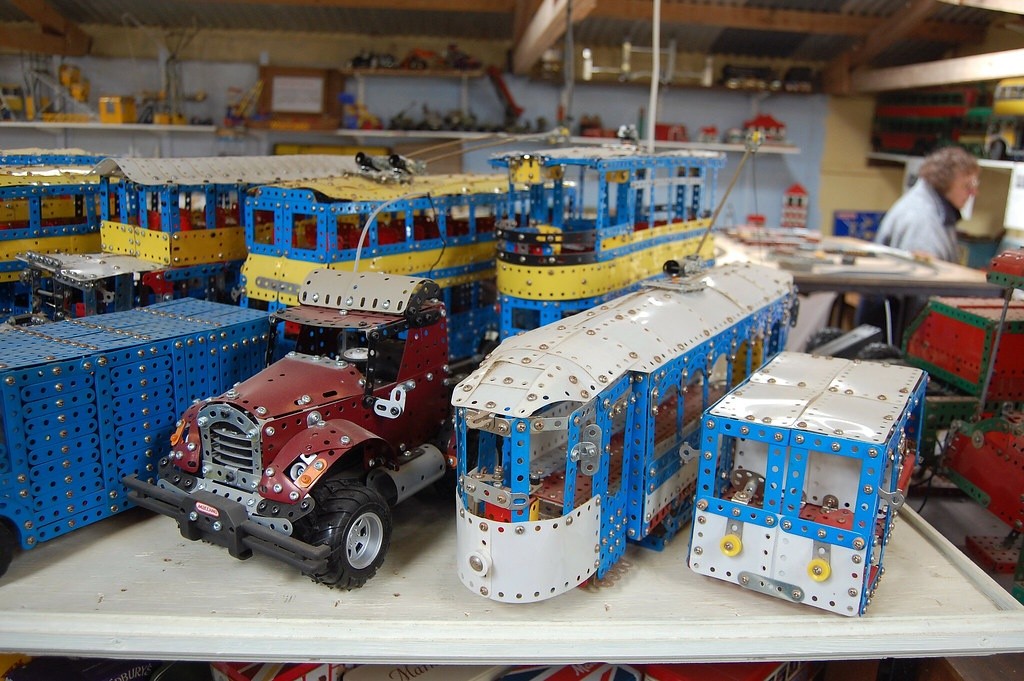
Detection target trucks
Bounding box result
[875,77,1024,166]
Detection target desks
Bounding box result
[0,484,1024,673]
[722,222,1013,351]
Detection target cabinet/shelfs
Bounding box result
[3,45,819,159]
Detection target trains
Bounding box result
[451,259,799,604]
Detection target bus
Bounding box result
[0,147,713,588]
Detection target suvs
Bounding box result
[122,266,477,597]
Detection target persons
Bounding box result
[853,145,981,351]
